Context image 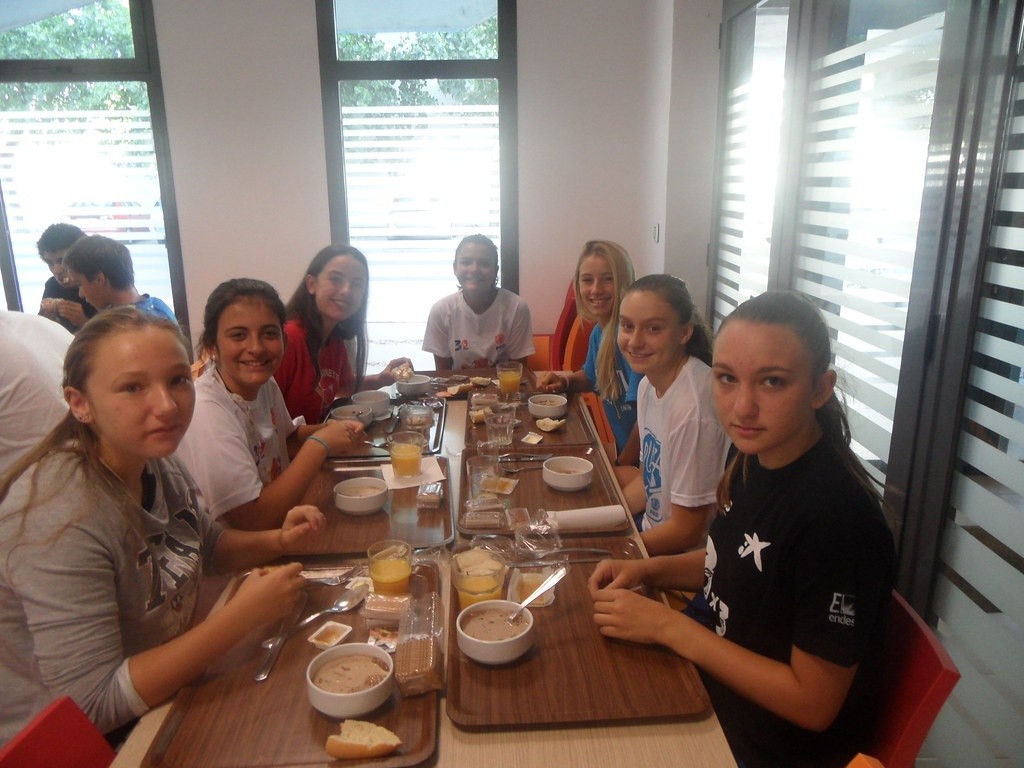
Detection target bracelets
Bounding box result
[307,435,331,455]
[553,373,570,393]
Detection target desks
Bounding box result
[111,368,735,766]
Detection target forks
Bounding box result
[516,543,613,561]
[306,562,367,587]
[364,440,387,448]
[502,465,543,473]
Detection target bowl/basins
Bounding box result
[333,476,388,517]
[351,389,390,417]
[542,455,594,493]
[528,394,568,419]
[396,375,431,398]
[306,641,394,720]
[456,599,535,666]
[330,404,373,428]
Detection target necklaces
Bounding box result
[213,366,233,394]
[657,356,687,397]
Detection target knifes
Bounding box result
[253,590,309,682]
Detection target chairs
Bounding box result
[0,696,117,768]
[809,590,964,768]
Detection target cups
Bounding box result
[482,403,516,447]
[387,431,425,480]
[496,361,523,396]
[451,549,506,611]
[466,454,500,502]
[367,539,412,597]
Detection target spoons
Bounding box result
[261,583,369,650]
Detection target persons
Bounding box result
[0,305,328,751]
[540,240,733,557]
[422,235,535,371]
[588,292,896,768]
[174,244,414,531]
[0,224,179,469]
